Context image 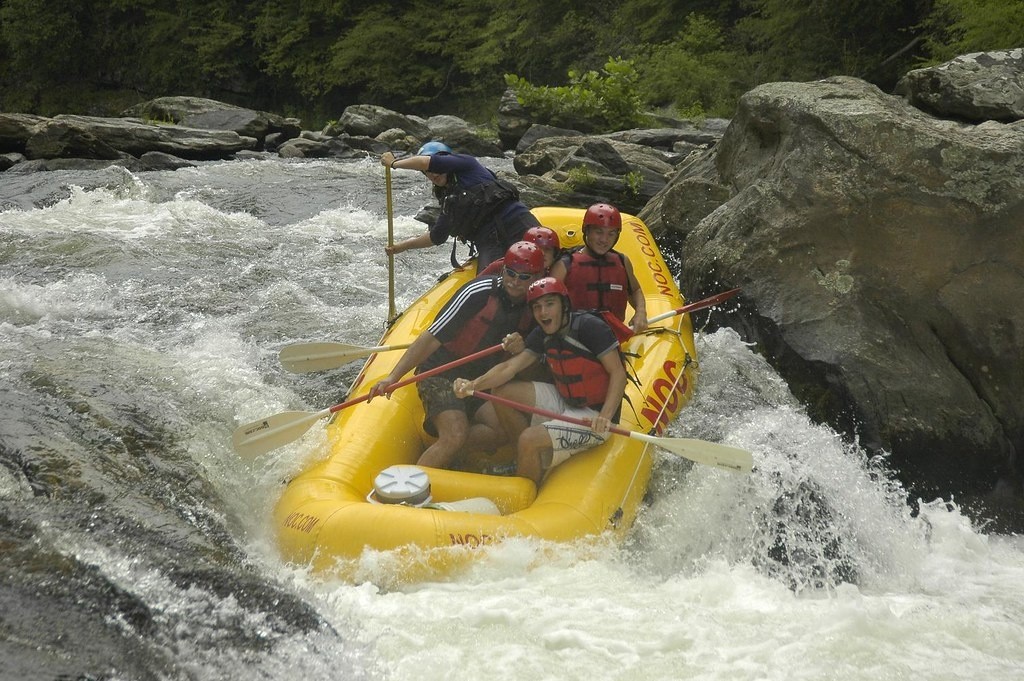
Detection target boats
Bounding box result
[270,207,697,594]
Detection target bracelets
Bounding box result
[390,160,399,169]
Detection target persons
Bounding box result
[544,203,649,337]
[366,242,543,471]
[452,277,629,485]
[380,140,542,276]
[475,223,561,277]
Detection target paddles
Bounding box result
[627,288,743,331]
[385,165,397,322]
[467,389,754,476]
[233,343,504,452]
[278,343,414,371]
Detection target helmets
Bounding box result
[417,142,452,175]
[504,241,546,274]
[526,277,572,307]
[524,226,560,249]
[582,204,622,234]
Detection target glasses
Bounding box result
[506,268,531,280]
